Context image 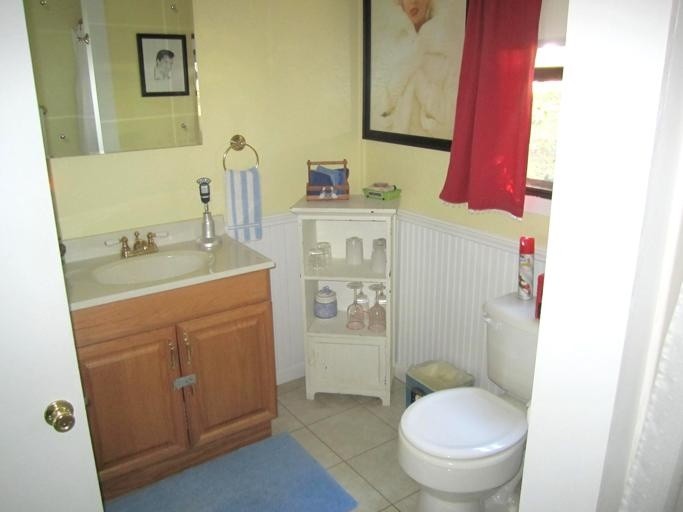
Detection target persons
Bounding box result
[151,47,176,81]
[382,0,437,128]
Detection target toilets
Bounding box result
[396,293,540,512]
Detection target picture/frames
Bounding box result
[135,33,189,97]
[362,0,464,152]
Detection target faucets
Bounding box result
[133,232,148,252]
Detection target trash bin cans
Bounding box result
[406,360,475,410]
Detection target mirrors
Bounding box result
[22,0,205,160]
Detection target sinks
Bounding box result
[93,248,216,285]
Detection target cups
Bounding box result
[346,237,363,265]
[310,241,332,272]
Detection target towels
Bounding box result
[225,168,264,245]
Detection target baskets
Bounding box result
[362,188,402,201]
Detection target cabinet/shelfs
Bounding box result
[286,191,402,405]
[81,301,278,477]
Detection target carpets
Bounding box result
[102,431,360,512]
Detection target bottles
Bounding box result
[313,287,339,320]
[370,238,387,279]
[517,236,534,303]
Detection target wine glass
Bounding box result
[368,283,386,333]
[346,282,365,330]
